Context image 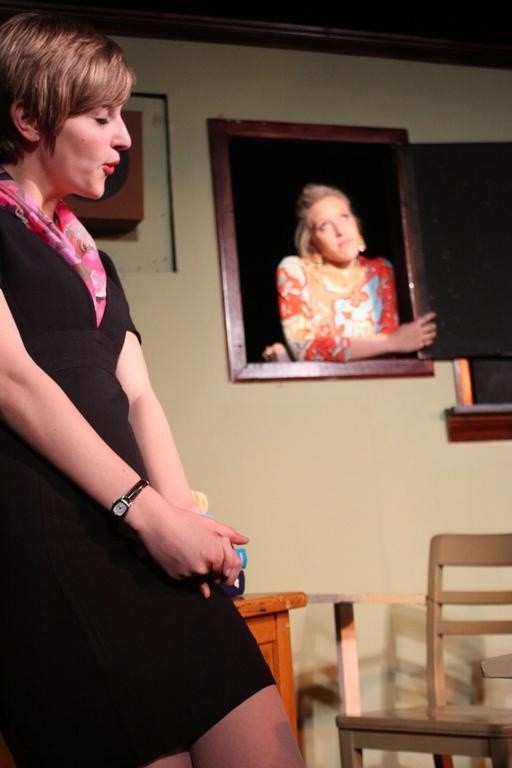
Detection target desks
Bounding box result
[235,592,307,745]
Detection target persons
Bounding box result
[260,182,440,364]
[0,10,308,768]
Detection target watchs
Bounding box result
[105,478,150,520]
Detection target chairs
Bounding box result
[382,600,489,768]
[335,534,512,768]
